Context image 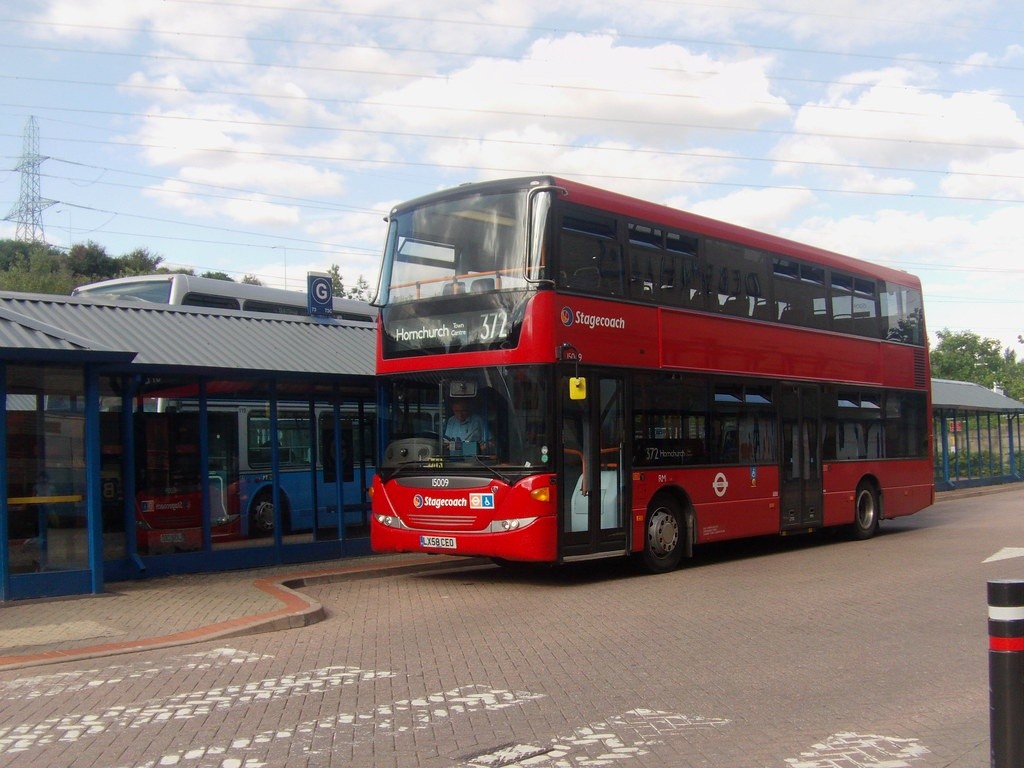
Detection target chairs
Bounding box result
[441,268,856,335]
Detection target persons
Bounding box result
[21,472,56,553]
[443,390,497,456]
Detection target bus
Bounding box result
[363,174,936,569]
[45,272,448,551]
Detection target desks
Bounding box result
[6,495,83,571]
